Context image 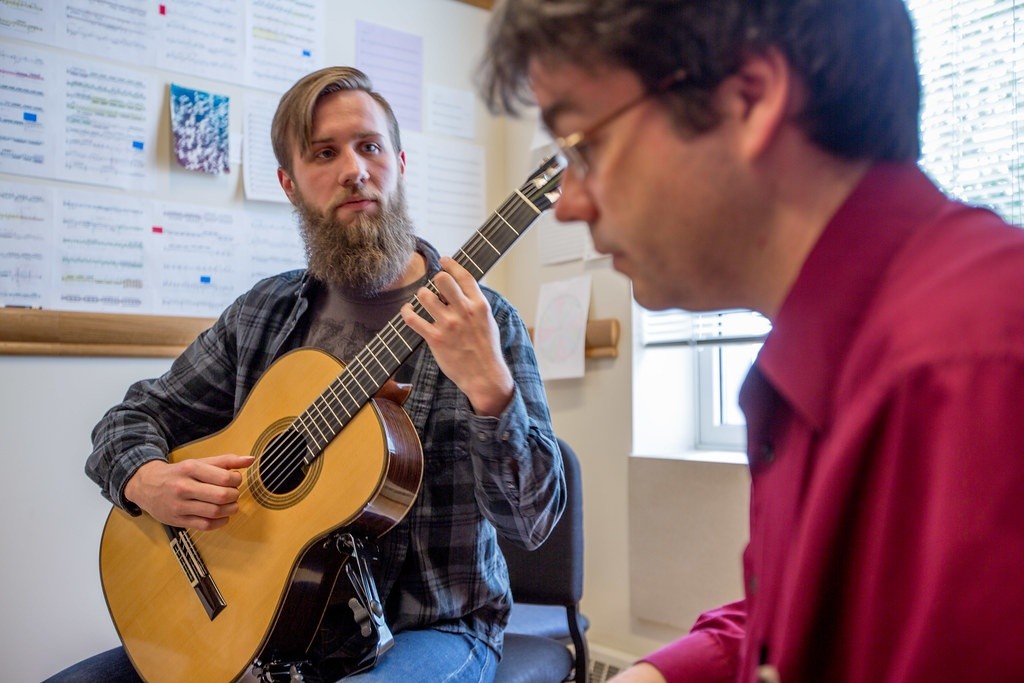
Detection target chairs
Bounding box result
[491,435,589,683]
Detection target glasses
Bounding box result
[554,24,792,179]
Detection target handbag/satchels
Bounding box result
[259,545,395,683]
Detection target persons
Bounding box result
[41,67,568,683]
[471,0,1024,683]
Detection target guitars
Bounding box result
[96,148,571,683]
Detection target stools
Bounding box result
[503,603,588,646]
